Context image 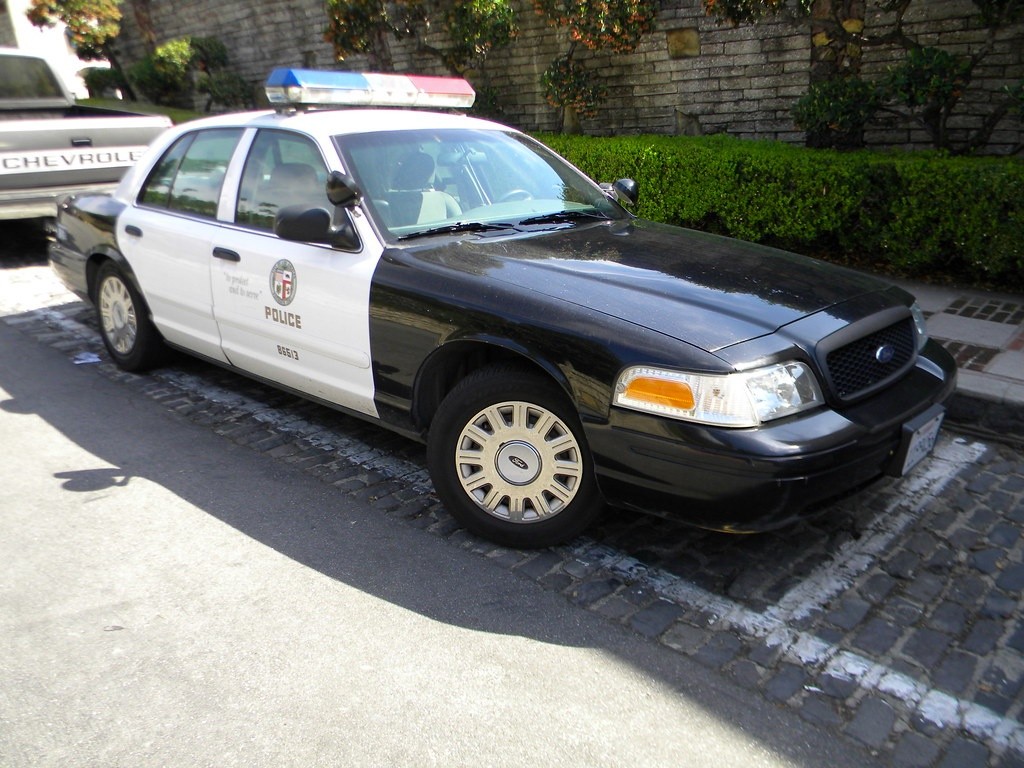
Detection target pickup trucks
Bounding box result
[0,44,176,223]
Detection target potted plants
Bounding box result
[76,67,121,98]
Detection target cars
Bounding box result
[44,64,959,552]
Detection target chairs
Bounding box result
[250,163,341,232]
[383,151,463,226]
